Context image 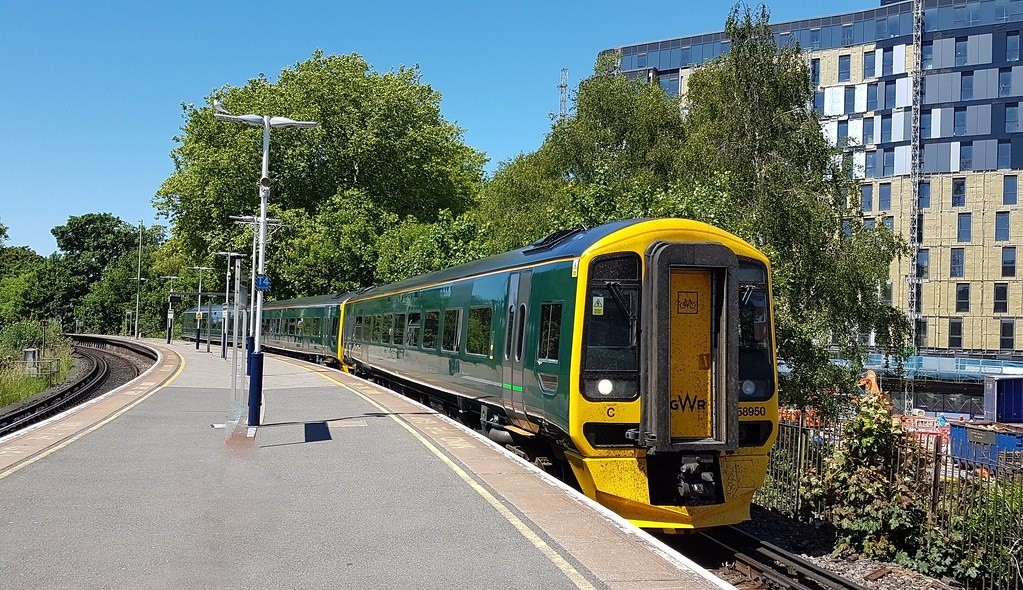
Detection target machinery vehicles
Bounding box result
[777,369,881,426]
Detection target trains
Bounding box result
[183,216,778,535]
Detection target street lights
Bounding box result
[228,214,282,376]
[134,218,144,340]
[186,266,214,350]
[214,113,319,427]
[215,252,248,359]
[160,276,179,345]
